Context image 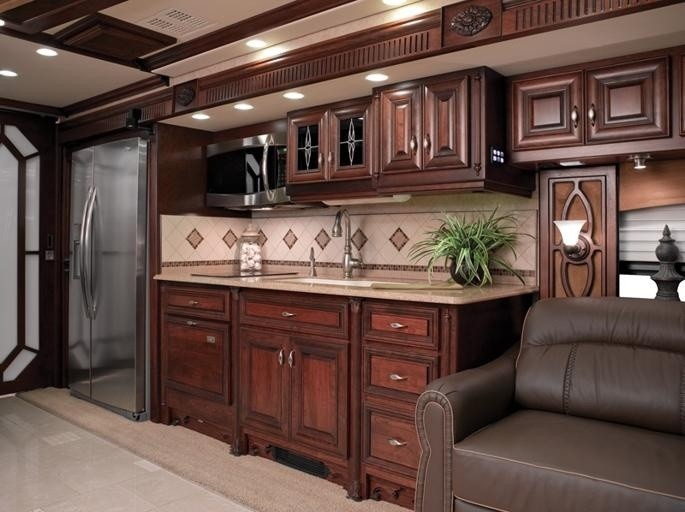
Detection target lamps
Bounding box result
[624,151,654,173]
[552,217,596,266]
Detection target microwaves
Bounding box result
[202,131,291,208]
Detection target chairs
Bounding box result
[409,293,684,511]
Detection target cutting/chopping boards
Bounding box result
[190,270,299,277]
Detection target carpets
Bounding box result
[15,384,414,511]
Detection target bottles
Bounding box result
[239,224,262,271]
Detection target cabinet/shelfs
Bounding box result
[357,289,539,511]
[239,276,357,504]
[371,65,538,200]
[150,273,239,456]
[287,94,395,200]
[509,44,683,161]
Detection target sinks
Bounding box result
[264,276,410,288]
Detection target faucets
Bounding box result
[309,247,317,277]
[332,208,364,279]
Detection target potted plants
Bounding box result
[407,204,536,289]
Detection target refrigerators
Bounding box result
[66,138,150,422]
